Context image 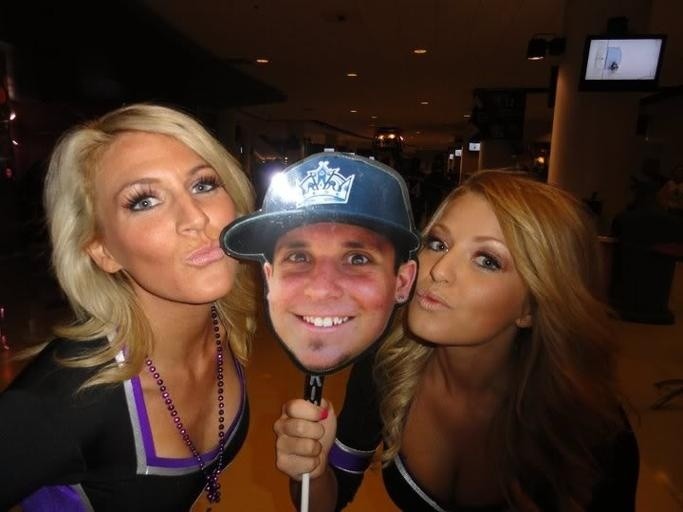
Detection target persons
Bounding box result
[219,149,422,376]
[0,104,261,512]
[273,170,641,511]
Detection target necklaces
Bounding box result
[144,306,223,512]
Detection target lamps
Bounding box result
[527,32,565,61]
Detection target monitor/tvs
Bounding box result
[583,33,667,90]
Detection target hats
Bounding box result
[220,150,422,264]
